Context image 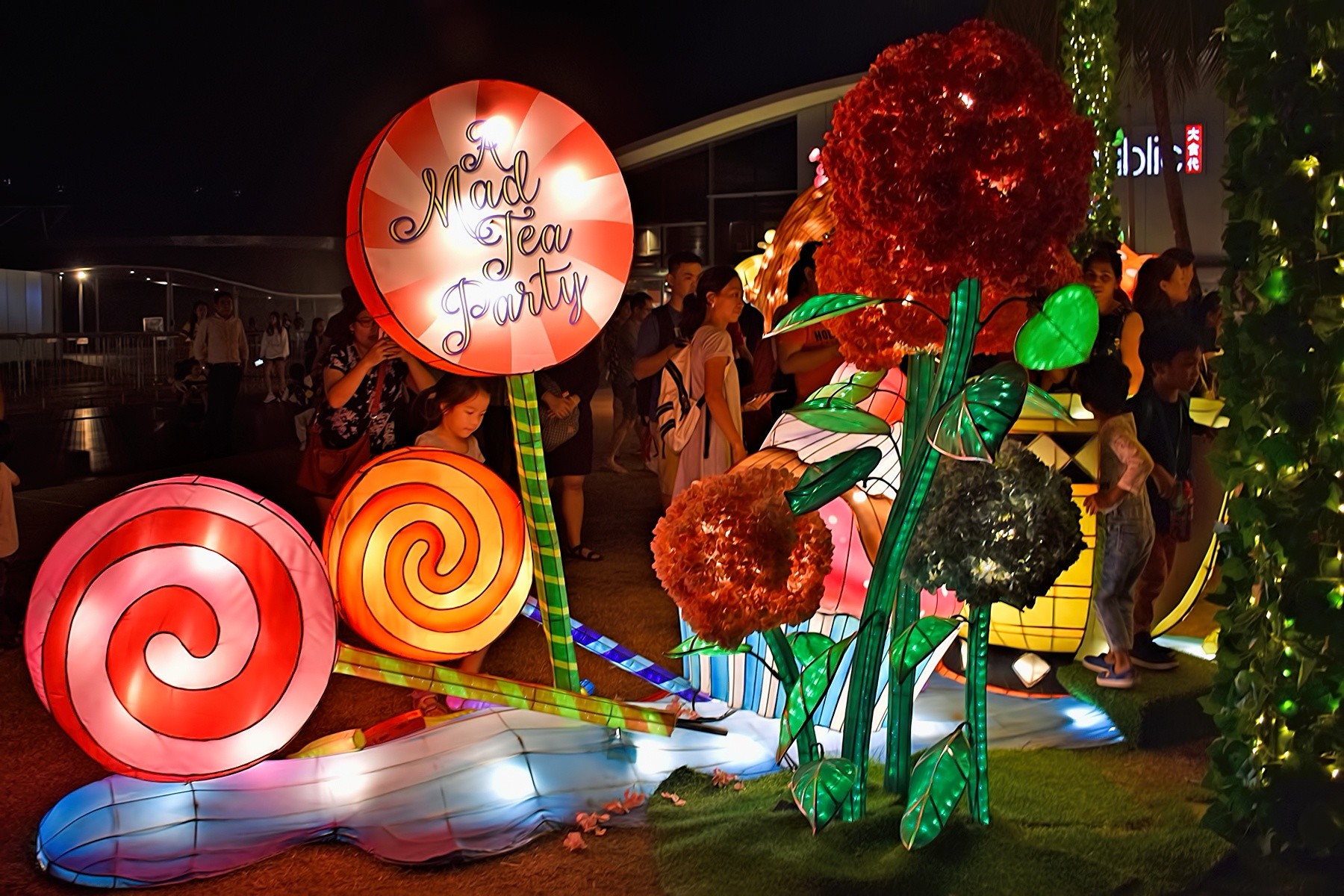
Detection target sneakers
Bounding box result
[264,395,275,402]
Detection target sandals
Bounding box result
[565,543,603,562]
[1096,664,1135,688]
[1083,653,1114,672]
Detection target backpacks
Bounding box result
[656,325,730,459]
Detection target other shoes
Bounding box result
[1130,642,1179,669]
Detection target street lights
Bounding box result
[75,272,86,335]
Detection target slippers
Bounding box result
[602,464,630,475]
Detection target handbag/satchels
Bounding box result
[542,405,580,452]
[295,423,371,500]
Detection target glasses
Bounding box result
[354,316,375,329]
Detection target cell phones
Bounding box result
[747,389,788,401]
[255,359,263,367]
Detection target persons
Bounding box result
[533,241,846,555]
[1043,245,1224,686]
[177,294,490,524]
